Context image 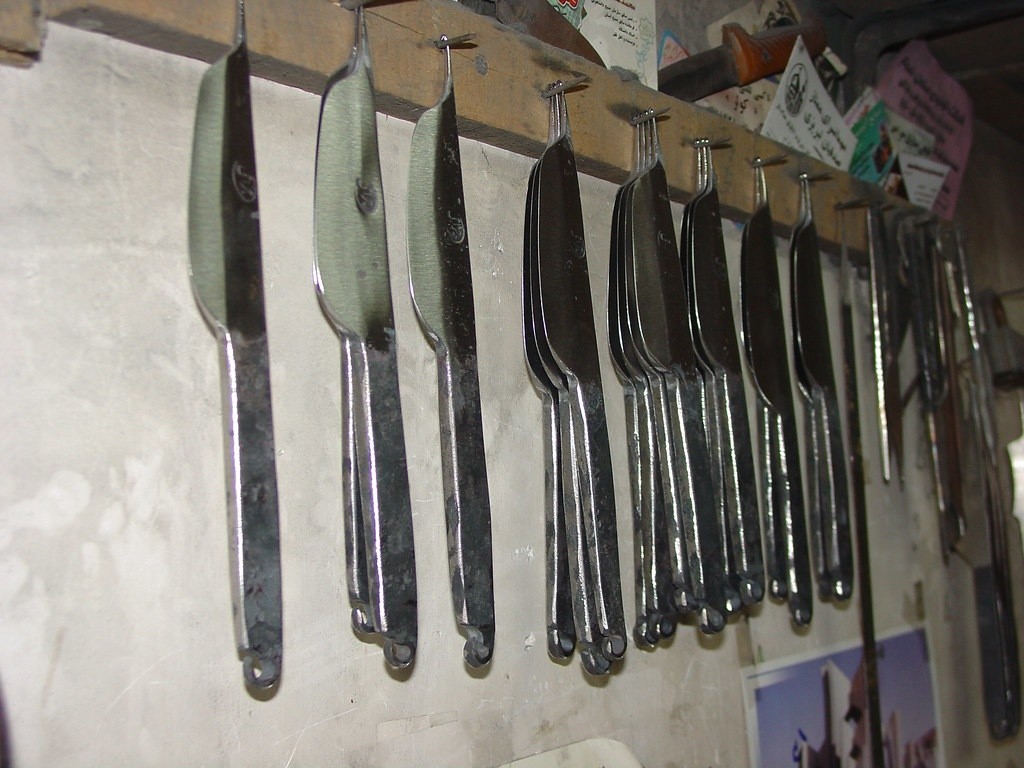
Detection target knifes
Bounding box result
[309,1,977,675]
[187,1,290,693]
